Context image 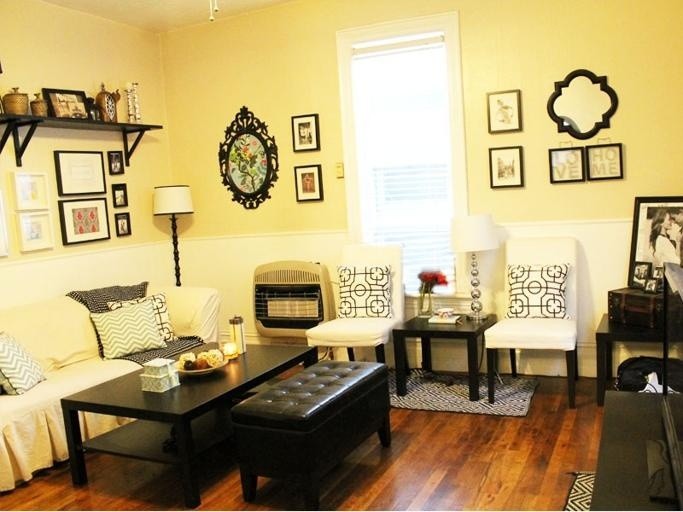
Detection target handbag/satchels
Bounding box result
[614,357,663,391]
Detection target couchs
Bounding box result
[0,283,220,497]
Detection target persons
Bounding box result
[668,206,683,268]
[649,208,681,279]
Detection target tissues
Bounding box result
[139,358,181,393]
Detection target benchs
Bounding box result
[228,359,393,511]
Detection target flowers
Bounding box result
[416,267,449,318]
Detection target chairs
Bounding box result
[306,241,404,363]
[484,234,579,408]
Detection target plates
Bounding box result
[176,359,229,375]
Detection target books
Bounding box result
[428,316,463,325]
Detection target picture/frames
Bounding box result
[631,260,652,288]
[488,145,524,189]
[218,106,279,209]
[548,147,584,183]
[585,143,623,182]
[644,279,657,294]
[291,113,321,154]
[293,163,326,203]
[485,89,523,135]
[628,196,683,292]
[41,87,89,123]
[0,150,133,261]
[653,267,665,292]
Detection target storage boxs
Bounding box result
[608,287,682,331]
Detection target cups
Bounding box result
[438,309,454,320]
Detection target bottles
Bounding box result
[229,315,246,354]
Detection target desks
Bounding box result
[588,391,683,509]
[596,314,682,406]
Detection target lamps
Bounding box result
[152,186,195,286]
[449,213,501,322]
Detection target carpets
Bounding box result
[383,369,540,419]
[560,468,596,510]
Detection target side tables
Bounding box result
[392,311,498,401]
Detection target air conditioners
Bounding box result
[252,261,328,340]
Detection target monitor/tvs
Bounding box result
[645,261,683,511]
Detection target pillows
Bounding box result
[504,263,569,320]
[335,263,393,318]
[106,293,179,344]
[90,299,165,359]
[0,329,48,396]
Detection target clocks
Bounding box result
[96,81,121,124]
